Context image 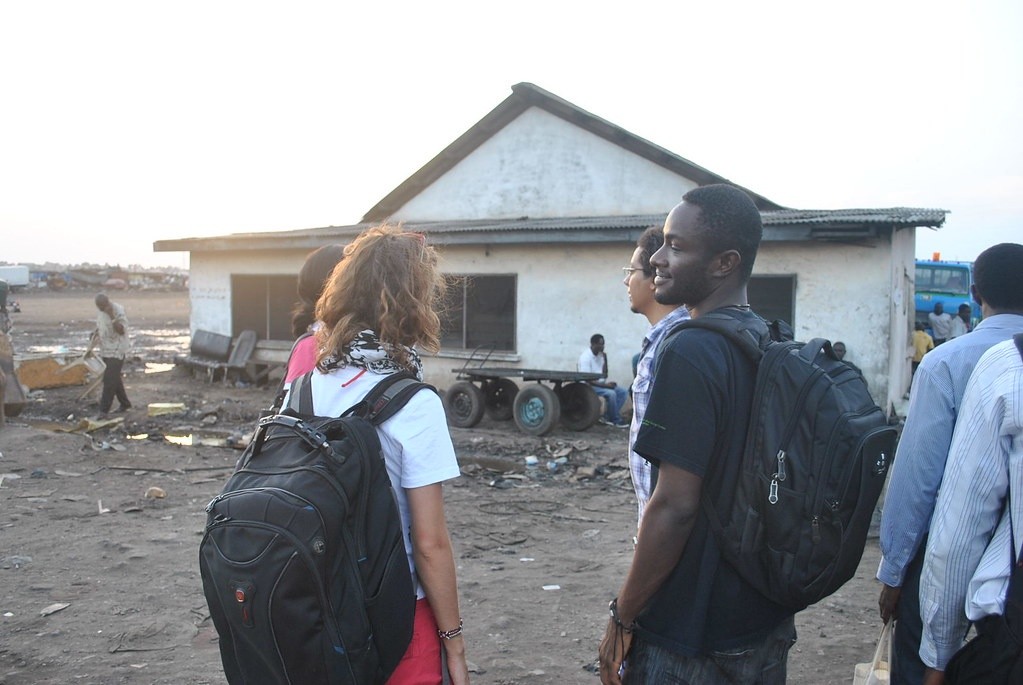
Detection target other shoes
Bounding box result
[598,416,615,426]
[615,420,630,429]
[96,411,107,421]
[113,405,133,413]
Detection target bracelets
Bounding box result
[112,319,116,323]
[438,618,463,639]
[610,598,635,668]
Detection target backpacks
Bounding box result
[650,311,899,610]
[198,366,442,683]
[1006,333,1023,650]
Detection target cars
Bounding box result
[105,278,129,290]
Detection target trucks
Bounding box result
[913,251,987,349]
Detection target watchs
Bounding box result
[632,536,638,551]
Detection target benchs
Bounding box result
[172,331,231,369]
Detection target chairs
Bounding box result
[218,330,256,385]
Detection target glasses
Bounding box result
[622,266,650,277]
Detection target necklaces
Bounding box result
[718,304,751,308]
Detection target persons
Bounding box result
[877,244,1023,685]
[598,184,797,685]
[283,227,472,685]
[946,304,971,342]
[928,302,952,347]
[577,334,631,428]
[89,293,132,420]
[623,225,690,553]
[912,323,934,373]
[0,280,15,429]
[832,342,846,360]
[628,338,649,401]
[284,243,348,395]
